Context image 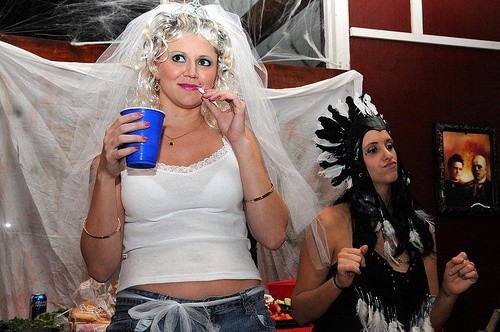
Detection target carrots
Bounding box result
[268,304,292,321]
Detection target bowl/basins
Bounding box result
[266,279,296,301]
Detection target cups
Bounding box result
[120,107,166,170]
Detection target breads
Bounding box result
[68,301,112,324]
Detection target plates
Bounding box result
[270,316,310,328]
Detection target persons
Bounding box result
[80,0,291,332]
[442,152,492,205]
[291,93,479,332]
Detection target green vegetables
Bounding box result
[0,307,69,332]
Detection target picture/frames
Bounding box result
[430,122,500,220]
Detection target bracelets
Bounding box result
[83,215,121,239]
[334,273,347,290]
[244,183,274,203]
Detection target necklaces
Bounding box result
[163,120,204,148]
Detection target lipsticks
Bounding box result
[195,87,205,94]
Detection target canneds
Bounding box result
[31,293,47,319]
